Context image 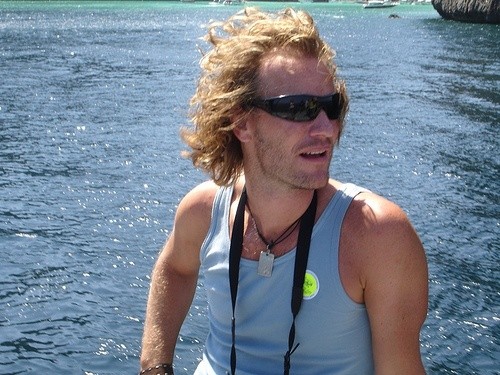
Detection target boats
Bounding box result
[364,0,396,8]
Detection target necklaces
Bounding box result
[246,201,301,278]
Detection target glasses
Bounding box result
[246,92,345,123]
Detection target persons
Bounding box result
[140,7,429,375]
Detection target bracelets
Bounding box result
[140,364,174,375]
